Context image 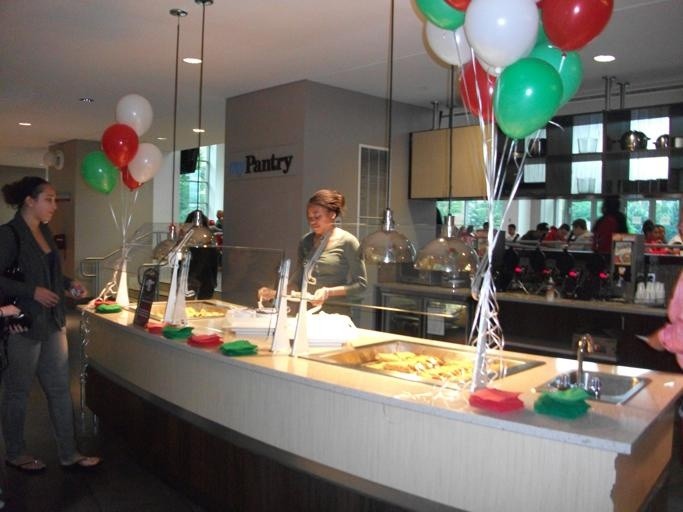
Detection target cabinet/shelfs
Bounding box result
[407,123,497,201]
[494,104,683,200]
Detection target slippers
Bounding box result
[59,456,103,469]
[5,457,48,475]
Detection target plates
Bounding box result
[286,294,313,302]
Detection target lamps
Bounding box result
[352,1,480,276]
[149,2,217,265]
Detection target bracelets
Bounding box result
[321,286,329,301]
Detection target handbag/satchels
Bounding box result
[1,261,33,335]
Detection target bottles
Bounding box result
[633,273,665,306]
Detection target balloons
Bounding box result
[115,93,154,139]
[412,1,614,143]
[120,167,145,191]
[101,123,140,168]
[127,142,163,186]
[78,149,120,195]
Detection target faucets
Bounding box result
[576,331,599,386]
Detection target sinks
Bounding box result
[534,368,652,406]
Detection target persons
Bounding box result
[625,207,681,374]
[1,175,105,473]
[0,304,31,336]
[593,194,628,255]
[477,217,681,260]
[214,209,223,229]
[257,189,369,326]
[174,210,217,300]
